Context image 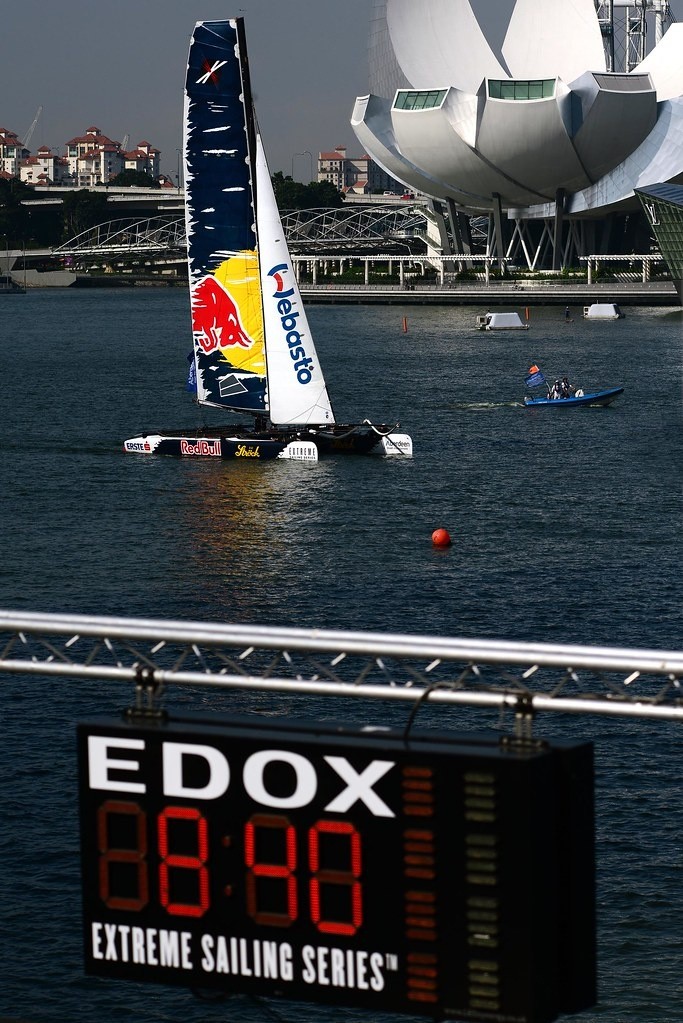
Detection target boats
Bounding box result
[524,358,625,409]
[581,302,625,321]
[474,312,531,331]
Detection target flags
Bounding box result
[529,365,540,374]
[524,371,546,388]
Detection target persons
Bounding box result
[561,377,575,399]
[565,306,570,320]
[551,380,562,399]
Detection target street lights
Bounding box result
[292,152,304,180]
[3,234,8,287]
[305,150,312,181]
[176,148,180,188]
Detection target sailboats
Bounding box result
[120,18,413,463]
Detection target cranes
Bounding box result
[20,104,43,149]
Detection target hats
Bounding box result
[563,377,568,380]
[555,380,559,382]
